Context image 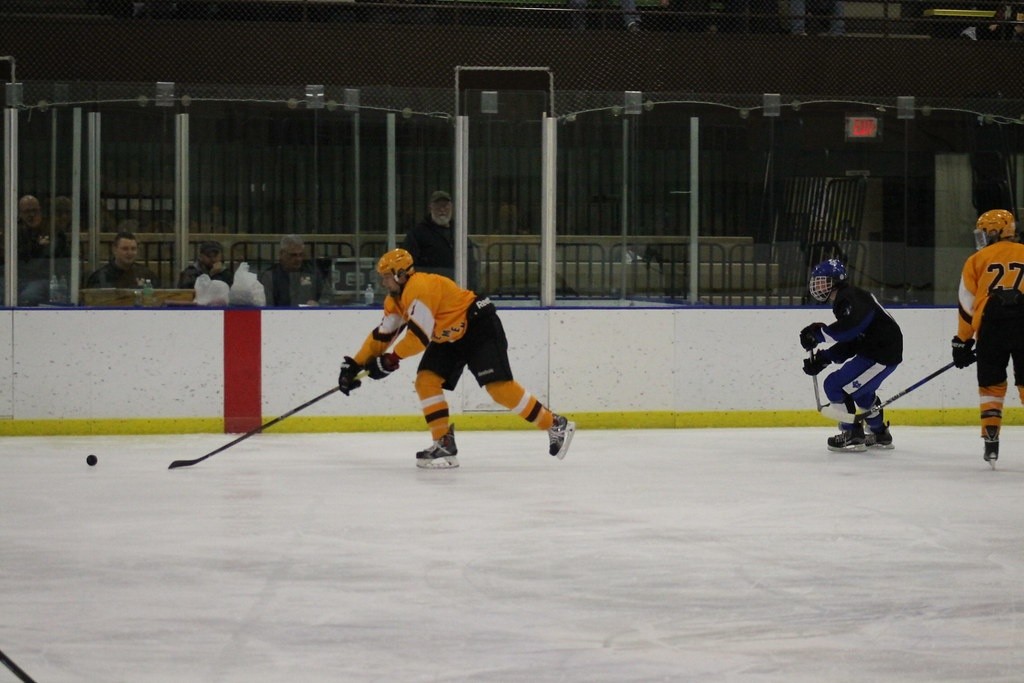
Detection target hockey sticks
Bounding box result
[809,346,831,411]
[168,371,370,469]
[820,348,976,424]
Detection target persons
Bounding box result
[952,209,1024,460]
[338,249,567,458]
[19,195,68,306]
[399,190,478,292]
[87,233,161,289]
[177,240,234,287]
[261,233,322,307]
[799,260,903,447]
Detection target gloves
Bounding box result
[365,353,399,380]
[338,356,361,397]
[951,336,978,370]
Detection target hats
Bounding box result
[200,242,221,254]
[430,190,453,202]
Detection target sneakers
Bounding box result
[799,322,824,352]
[548,412,577,461]
[416,423,460,470]
[826,432,868,453]
[983,439,1000,470]
[802,349,831,376]
[865,425,895,449]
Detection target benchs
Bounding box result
[64,231,792,290]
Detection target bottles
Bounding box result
[143,279,154,306]
[365,284,374,305]
[49,276,59,302]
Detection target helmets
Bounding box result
[974,209,1016,240]
[809,259,850,303]
[377,249,415,286]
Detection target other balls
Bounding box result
[87,455,97,466]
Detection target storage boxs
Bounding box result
[316,255,380,294]
